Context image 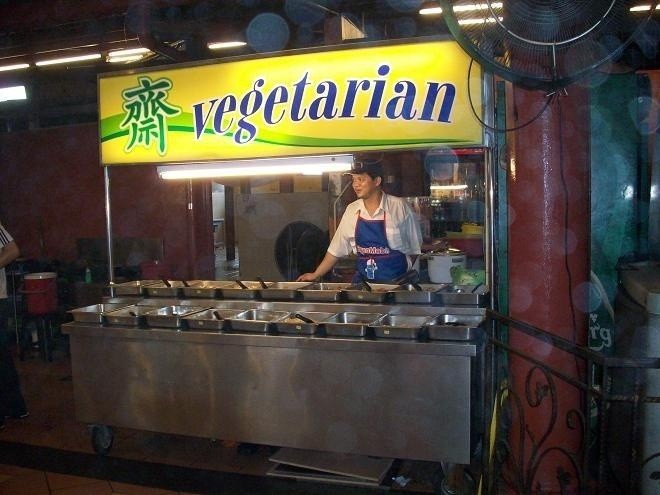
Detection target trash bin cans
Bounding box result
[606,258,660,495]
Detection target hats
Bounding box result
[342,159,383,178]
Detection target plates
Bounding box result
[424,315,486,340]
[181,307,246,330]
[297,283,359,303]
[138,306,211,329]
[385,283,447,304]
[270,311,337,334]
[139,279,201,298]
[338,283,400,304]
[106,279,162,296]
[368,314,437,340]
[432,284,486,305]
[217,280,273,300]
[68,303,136,323]
[319,312,387,337]
[178,278,222,300]
[254,281,316,301]
[222,308,290,334]
[100,304,164,326]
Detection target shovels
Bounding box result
[392,269,422,291]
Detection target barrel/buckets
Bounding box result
[19,271,58,315]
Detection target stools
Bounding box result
[19,313,58,362]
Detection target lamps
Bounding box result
[155,154,356,180]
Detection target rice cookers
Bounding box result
[426,247,468,282]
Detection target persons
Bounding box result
[0,224,30,433]
[296,156,424,283]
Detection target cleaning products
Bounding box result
[85,268,92,282]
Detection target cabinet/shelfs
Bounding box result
[61,276,492,464]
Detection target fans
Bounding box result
[439,0,660,99]
